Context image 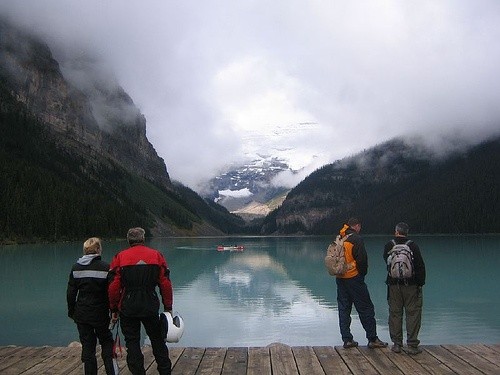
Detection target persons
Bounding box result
[107,226,173,375]
[383,222,426,355]
[65,237,110,375]
[336,217,389,348]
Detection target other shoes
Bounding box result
[408,346,423,355]
[388,344,401,352]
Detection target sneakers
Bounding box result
[343,339,358,348]
[367,338,388,348]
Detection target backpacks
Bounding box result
[325,232,356,275]
[385,238,417,281]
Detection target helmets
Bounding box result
[160,312,184,343]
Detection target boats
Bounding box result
[217,244,244,252]
